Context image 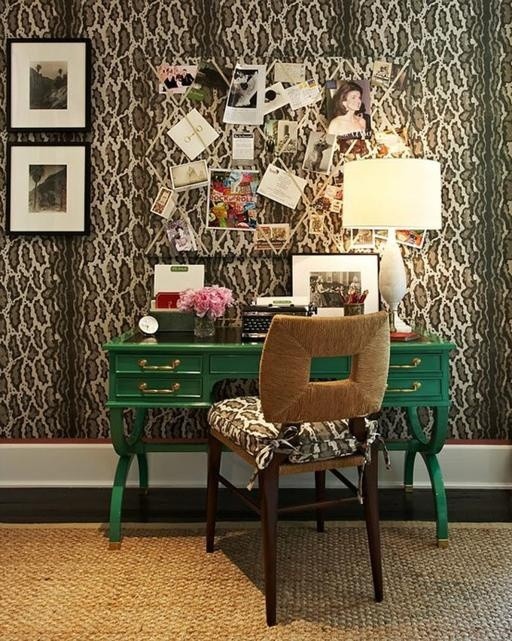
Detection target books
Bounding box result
[388,330,421,343]
[152,260,208,301]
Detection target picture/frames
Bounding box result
[6,141,90,236]
[290,253,381,316]
[6,38,91,133]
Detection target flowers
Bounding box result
[177,284,235,321]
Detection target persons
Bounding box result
[329,82,367,140]
[349,278,360,292]
[313,278,327,308]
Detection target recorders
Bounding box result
[241,304,317,342]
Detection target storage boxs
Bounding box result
[149,264,204,331]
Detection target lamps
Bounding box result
[343,159,441,342]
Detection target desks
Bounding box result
[102,327,457,550]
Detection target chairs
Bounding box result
[206,312,390,625]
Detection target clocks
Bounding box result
[138,312,160,337]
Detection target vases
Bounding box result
[193,311,216,337]
